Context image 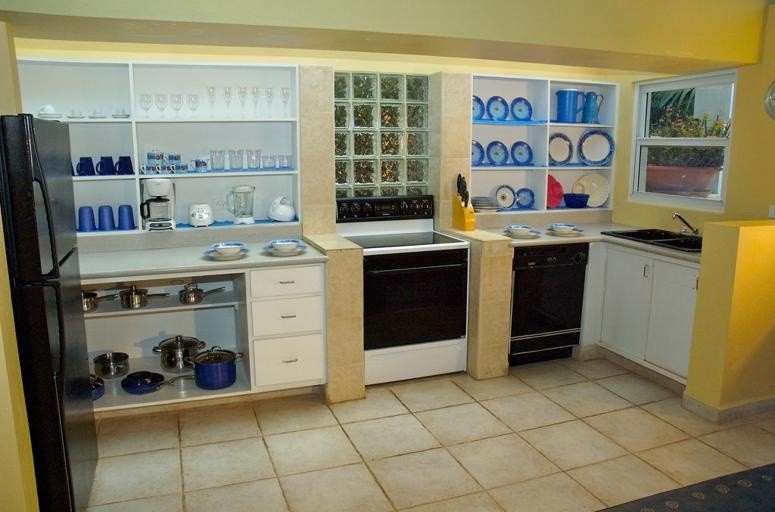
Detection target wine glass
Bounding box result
[154,93,169,112]
[224,87,233,108]
[264,87,274,108]
[139,94,153,113]
[186,94,199,111]
[281,88,289,109]
[207,86,217,108]
[251,87,260,108]
[238,86,248,108]
[170,94,184,117]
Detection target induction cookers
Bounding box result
[344,232,465,249]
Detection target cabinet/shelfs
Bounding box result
[547,78,619,213]
[245,240,329,392]
[336,69,429,199]
[78,244,250,413]
[142,61,301,234]
[601,247,702,388]
[15,58,142,233]
[470,73,550,214]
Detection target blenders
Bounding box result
[140,178,176,231]
[225,185,255,225]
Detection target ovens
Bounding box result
[363,247,468,351]
[509,242,590,355]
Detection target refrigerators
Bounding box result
[1,112,99,512]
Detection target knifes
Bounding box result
[457,173,469,208]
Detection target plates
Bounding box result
[202,249,250,261]
[572,173,610,208]
[38,113,62,118]
[112,114,129,118]
[511,141,533,166]
[471,196,504,212]
[472,95,485,120]
[501,230,544,238]
[547,175,563,208]
[549,133,574,166]
[576,128,616,166]
[88,116,106,119]
[67,116,85,119]
[263,245,308,256]
[544,228,584,237]
[471,140,485,166]
[496,185,518,210]
[516,188,535,209]
[511,97,532,121]
[486,96,509,121]
[486,141,509,166]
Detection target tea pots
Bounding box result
[582,92,603,124]
[555,89,585,123]
[267,194,296,222]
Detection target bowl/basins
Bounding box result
[211,241,247,256]
[504,224,535,234]
[550,223,576,232]
[564,193,589,208]
[269,239,301,253]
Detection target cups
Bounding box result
[190,158,210,172]
[98,205,115,231]
[209,150,226,170]
[78,206,96,232]
[228,148,244,169]
[170,162,188,173]
[163,152,181,164]
[96,156,115,175]
[139,164,158,174]
[93,108,103,116]
[38,103,57,114]
[76,157,95,176]
[115,107,125,114]
[71,109,81,116]
[246,149,262,169]
[114,156,133,175]
[118,204,135,230]
[262,152,277,170]
[277,152,294,167]
[155,163,172,174]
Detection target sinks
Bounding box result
[654,238,702,250]
[613,229,683,241]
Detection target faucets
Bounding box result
[672,212,698,234]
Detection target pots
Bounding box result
[153,335,206,374]
[119,285,170,309]
[89,374,105,401]
[179,283,226,305]
[184,346,245,390]
[81,290,119,313]
[93,351,129,379]
[121,370,195,395]
